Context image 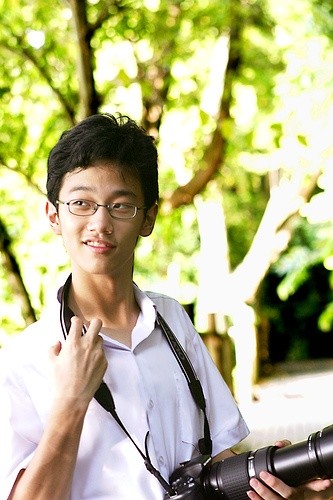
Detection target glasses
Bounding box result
[56,200,147,219]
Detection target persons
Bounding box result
[0,114,333,500]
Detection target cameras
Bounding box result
[164,424,333,500]
